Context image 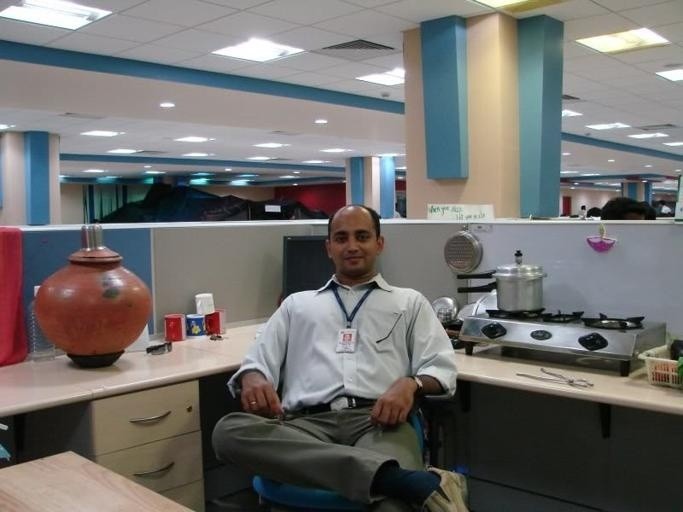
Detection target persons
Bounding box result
[212,204,470,512]
[587,198,673,220]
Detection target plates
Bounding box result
[65,350,124,368]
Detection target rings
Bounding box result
[249,401,256,405]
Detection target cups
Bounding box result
[164,293,226,341]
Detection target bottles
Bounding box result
[28,285,56,362]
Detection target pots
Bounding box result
[456,249,546,314]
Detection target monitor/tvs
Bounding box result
[282,235,336,297]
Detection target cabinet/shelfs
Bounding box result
[31,379,206,512]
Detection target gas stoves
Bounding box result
[457,308,665,377]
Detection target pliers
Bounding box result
[515,367,594,388]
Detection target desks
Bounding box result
[152,318,268,358]
[1,343,239,466]
[453,342,683,438]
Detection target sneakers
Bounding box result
[419,466,469,512]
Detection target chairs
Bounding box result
[252,386,447,512]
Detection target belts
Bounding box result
[300,395,377,416]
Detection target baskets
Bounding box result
[638,344,682,390]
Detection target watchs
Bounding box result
[407,375,423,390]
